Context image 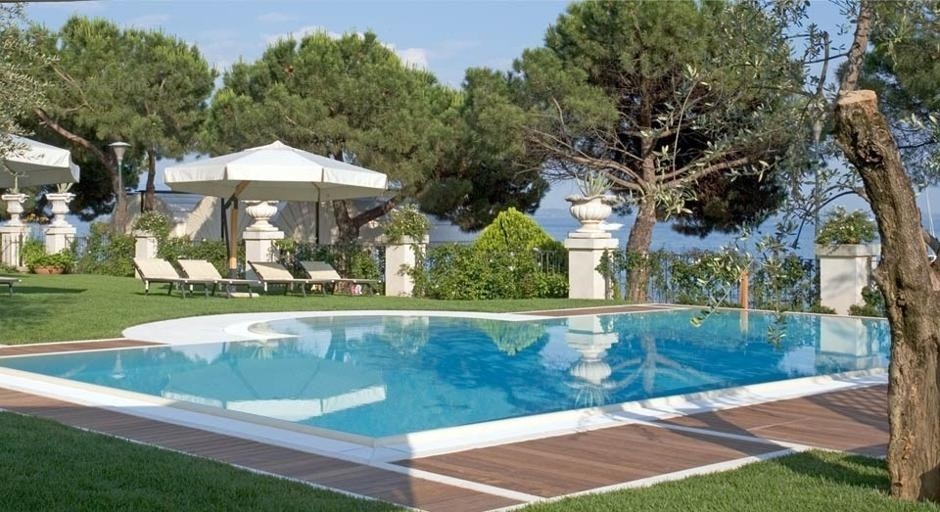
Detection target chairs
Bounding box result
[131,256,381,301]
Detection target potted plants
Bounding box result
[21,238,79,275]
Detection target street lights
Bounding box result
[109,140,132,234]
[806,101,827,312]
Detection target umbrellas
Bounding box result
[162,139,390,292]
[0,130,81,195]
[160,337,389,425]
